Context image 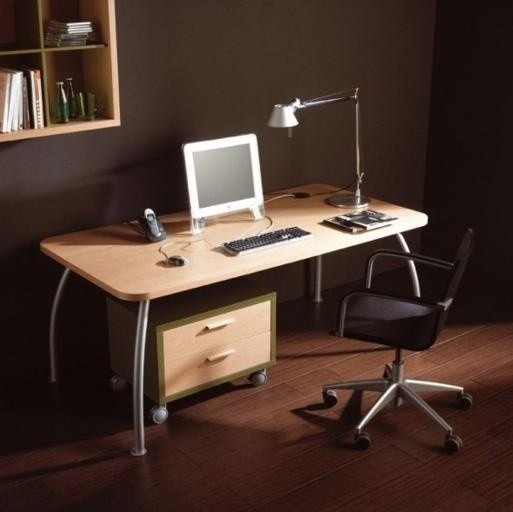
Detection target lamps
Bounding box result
[268,86,370,210]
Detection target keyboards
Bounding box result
[223,227,312,255]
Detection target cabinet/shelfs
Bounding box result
[0,0,121,146]
[104,280,279,424]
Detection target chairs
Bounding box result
[323,227,476,457]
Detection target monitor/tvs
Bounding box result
[181,133,266,236]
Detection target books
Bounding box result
[44,20,94,48]
[324,217,392,234]
[0,67,45,133]
[336,208,399,231]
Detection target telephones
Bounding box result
[144,208,166,241]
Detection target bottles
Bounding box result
[53,81,70,123]
[65,77,78,117]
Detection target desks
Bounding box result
[23,183,429,460]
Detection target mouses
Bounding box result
[168,254,187,266]
